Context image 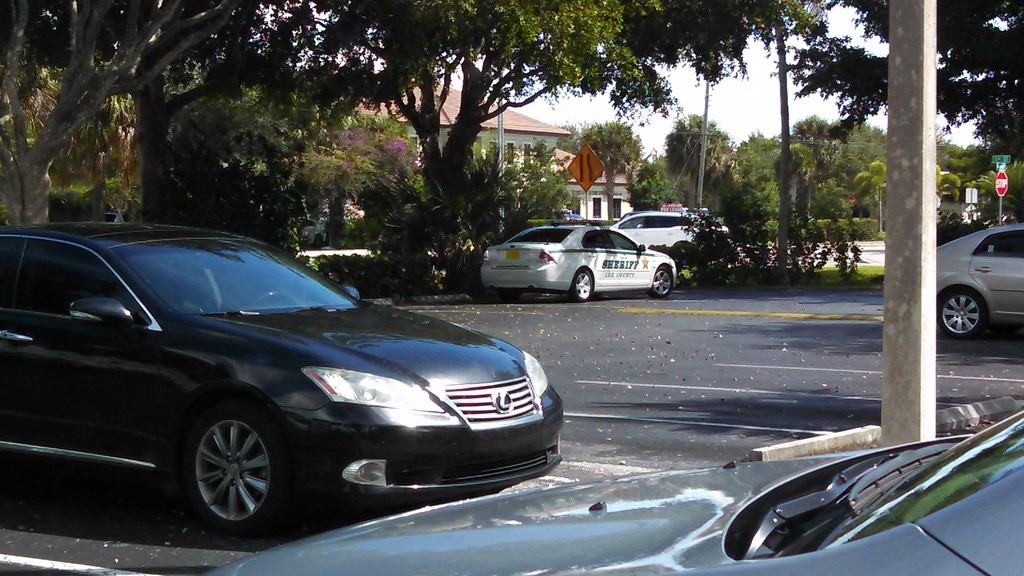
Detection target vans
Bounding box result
[609,211,697,248]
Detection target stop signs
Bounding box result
[995,171,1008,196]
[847,196,855,207]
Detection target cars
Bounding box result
[0,221,565,540]
[564,214,583,221]
[201,415,1024,576]
[481,225,677,302]
[935,223,1024,339]
